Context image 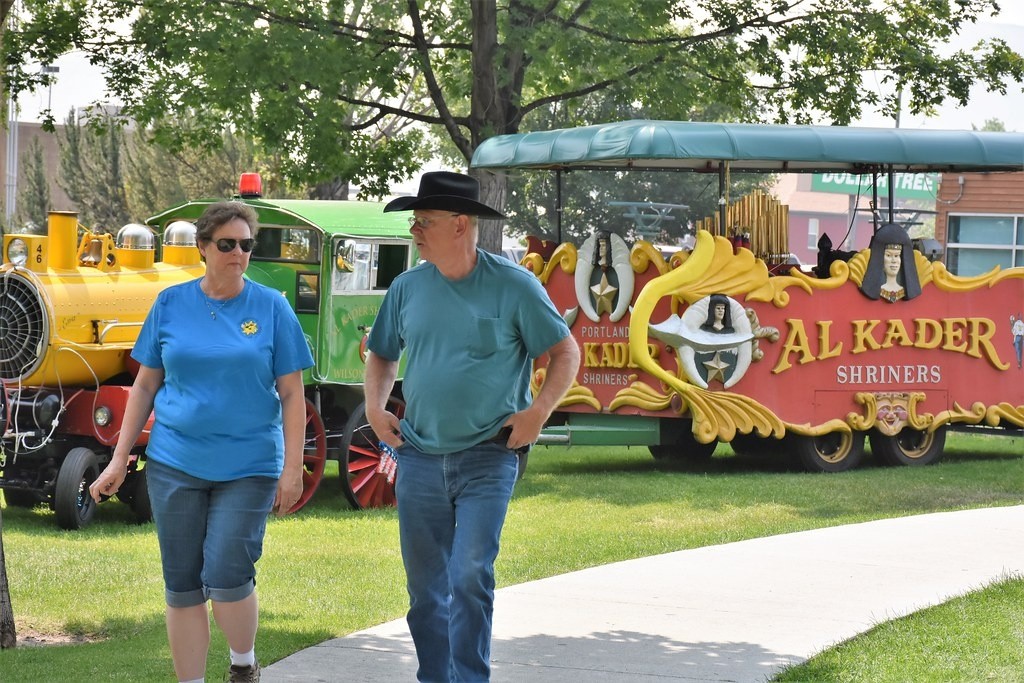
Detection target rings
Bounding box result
[293,500,298,503]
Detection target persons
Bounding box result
[364,171,580,683]
[89,202,316,683]
[698,293,736,335]
[860,224,923,301]
[591,231,614,269]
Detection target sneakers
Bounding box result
[228,654,260,683]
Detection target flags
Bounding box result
[377,441,398,485]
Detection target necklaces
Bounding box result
[202,278,243,321]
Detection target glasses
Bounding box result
[207,237,256,253]
[408,213,458,228]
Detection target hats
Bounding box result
[383,171,508,220]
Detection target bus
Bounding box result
[465,118,1023,477]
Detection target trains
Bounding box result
[2,165,425,534]
